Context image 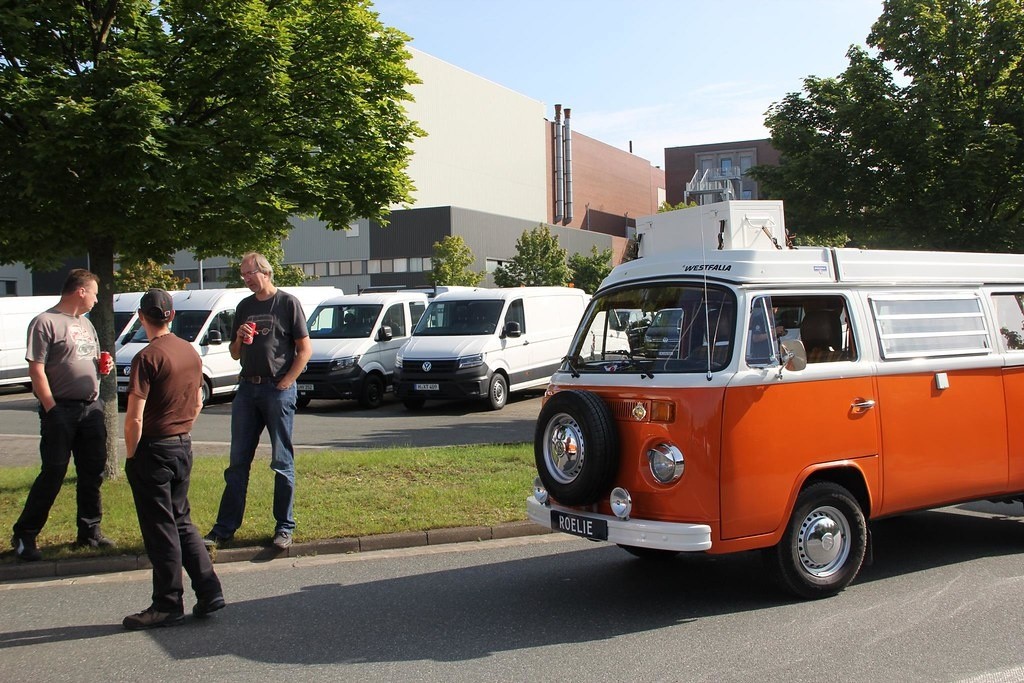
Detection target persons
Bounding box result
[746,301,785,366]
[9,271,117,562]
[121,287,227,631]
[201,251,314,551]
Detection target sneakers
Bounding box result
[272,531,292,549]
[11,533,43,561]
[192,596,225,616]
[76,535,117,548]
[202,537,233,547]
[123,604,185,629]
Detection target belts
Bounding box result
[243,374,274,385]
[146,434,189,441]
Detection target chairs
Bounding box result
[680,298,735,368]
[784,302,852,364]
[816,363,817,368]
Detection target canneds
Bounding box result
[243,322,256,344]
[99,352,110,374]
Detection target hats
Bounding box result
[141,287,173,322]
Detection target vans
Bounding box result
[0,285,684,414]
[523,249,1024,601]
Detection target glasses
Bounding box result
[240,269,259,280]
